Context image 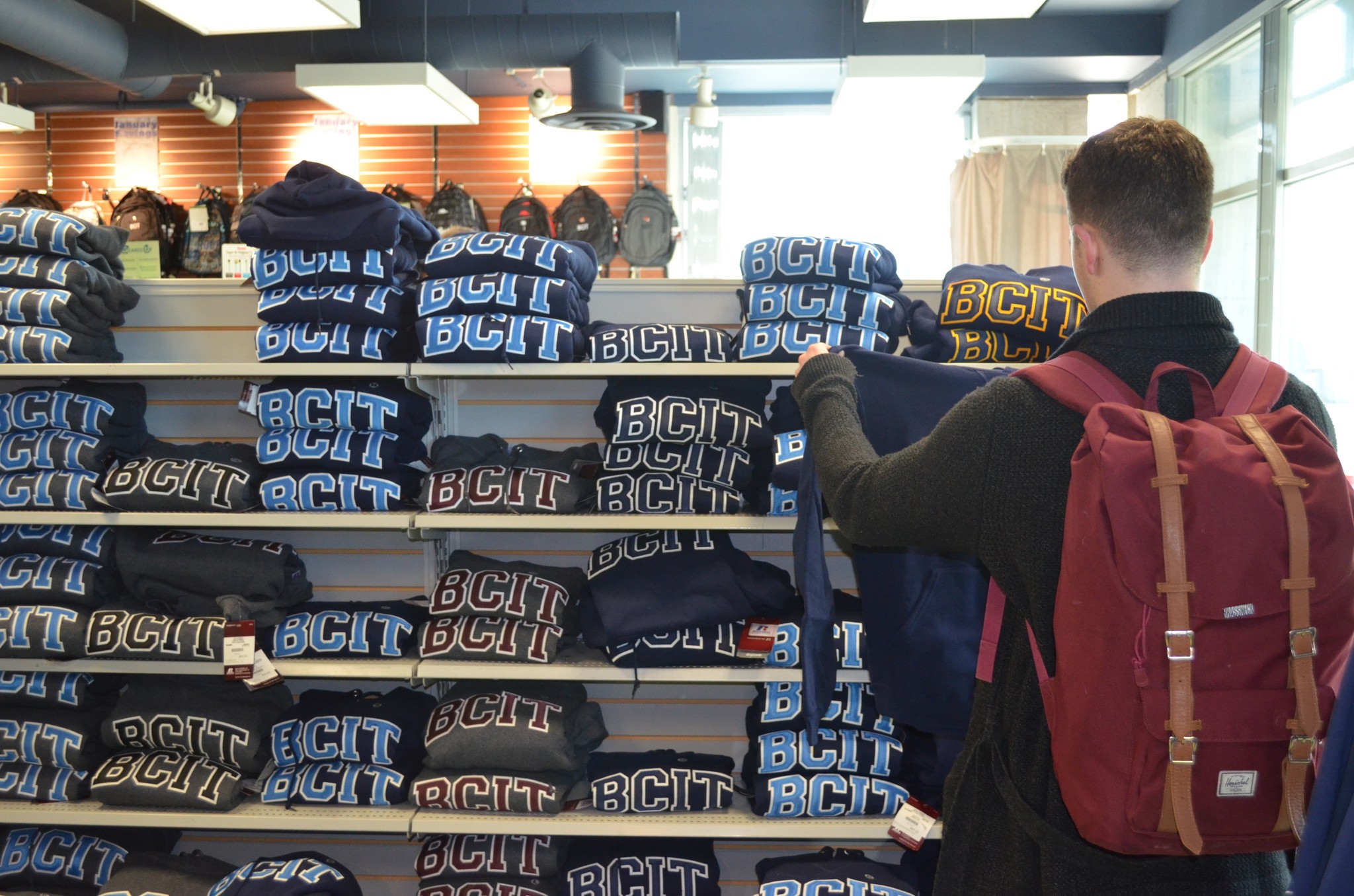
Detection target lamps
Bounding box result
[136,0,363,37]
[828,51,991,118]
[526,86,556,122]
[0,80,37,134]
[859,0,1051,27]
[688,72,723,132]
[290,59,482,130]
[181,72,242,133]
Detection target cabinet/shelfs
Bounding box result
[0,275,1050,896]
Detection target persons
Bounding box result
[791,118,1337,896]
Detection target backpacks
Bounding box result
[1,190,63,212]
[230,185,266,244]
[551,185,618,265]
[62,187,106,225]
[424,179,489,238]
[500,186,551,239]
[1007,344,1354,855]
[380,184,414,209]
[176,187,235,276]
[110,189,181,278]
[617,183,681,268]
